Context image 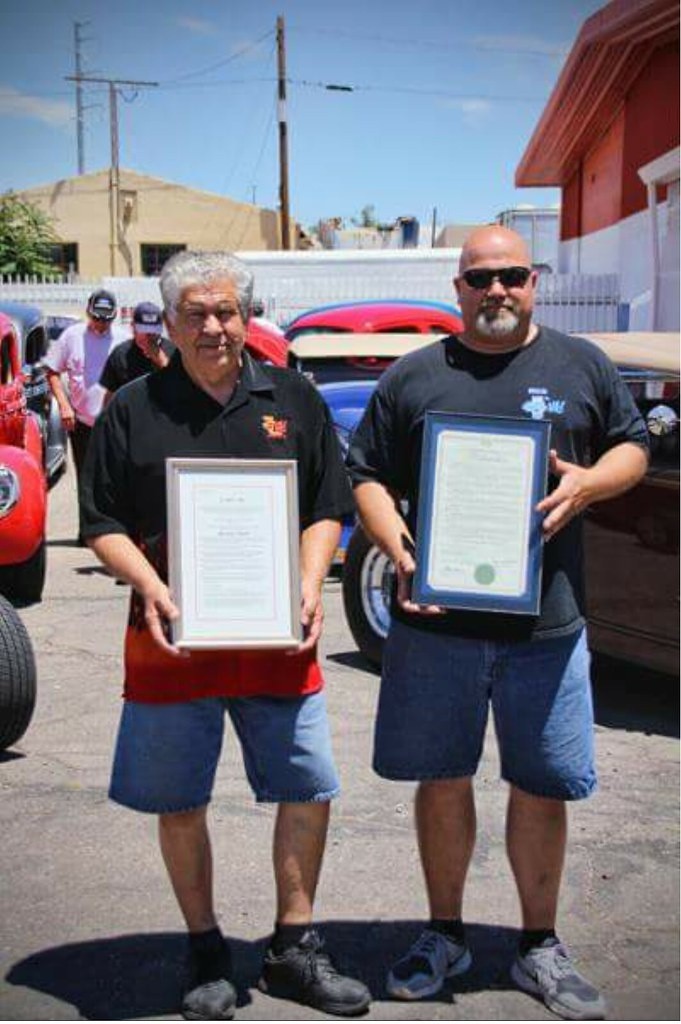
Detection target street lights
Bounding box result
[276,77,354,251]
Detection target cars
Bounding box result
[41,310,85,353]
[287,333,453,384]
[0,311,48,607]
[340,332,681,680]
[0,300,69,481]
[305,379,435,568]
[573,463,576,472]
[279,297,464,330]
[0,591,38,750]
[283,303,466,342]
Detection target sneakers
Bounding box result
[387,928,470,999]
[510,936,608,1019]
[183,948,238,1019]
[258,930,372,1015]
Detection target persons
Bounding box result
[39,291,135,548]
[346,224,653,1020]
[97,301,182,409]
[80,251,368,1021]
[248,297,286,337]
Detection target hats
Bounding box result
[89,291,116,319]
[134,302,164,335]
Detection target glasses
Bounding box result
[455,267,530,289]
